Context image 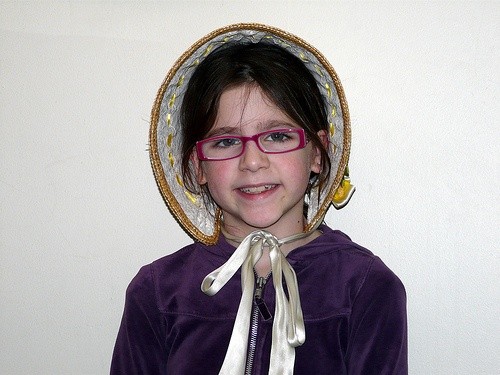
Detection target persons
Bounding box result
[109,23,408,375]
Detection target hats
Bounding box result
[147,22,351,247]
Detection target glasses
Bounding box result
[195,128,311,160]
